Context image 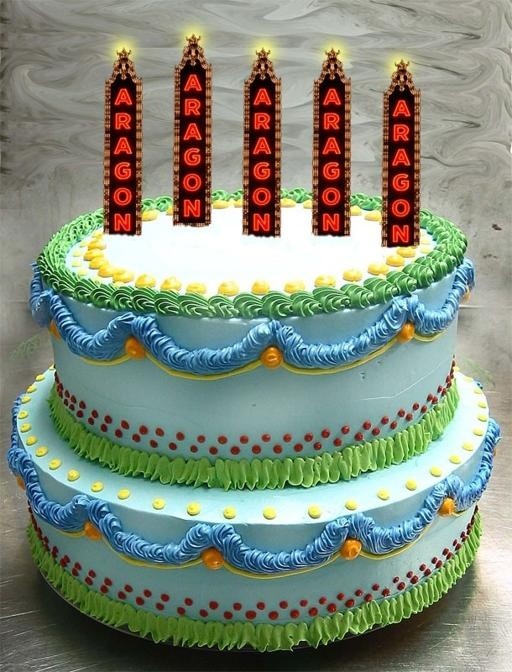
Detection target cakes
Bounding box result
[7,188,501,652]
